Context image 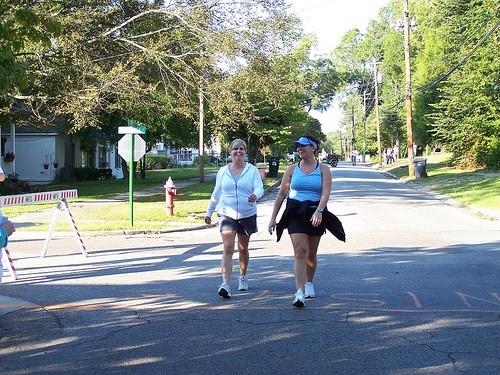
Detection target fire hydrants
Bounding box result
[163,176,177,216]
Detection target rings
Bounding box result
[253,197,256,200]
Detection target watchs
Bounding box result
[316,207,324,213]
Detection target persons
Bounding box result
[350,147,358,167]
[203,139,265,299]
[267,133,347,307]
[0,161,16,309]
[385,146,396,164]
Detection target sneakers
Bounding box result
[293,290,306,307]
[305,281,316,298]
[218,283,232,298]
[238,275,249,291]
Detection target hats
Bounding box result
[292,136,318,150]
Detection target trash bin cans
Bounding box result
[412,158,428,179]
[267,158,280,176]
